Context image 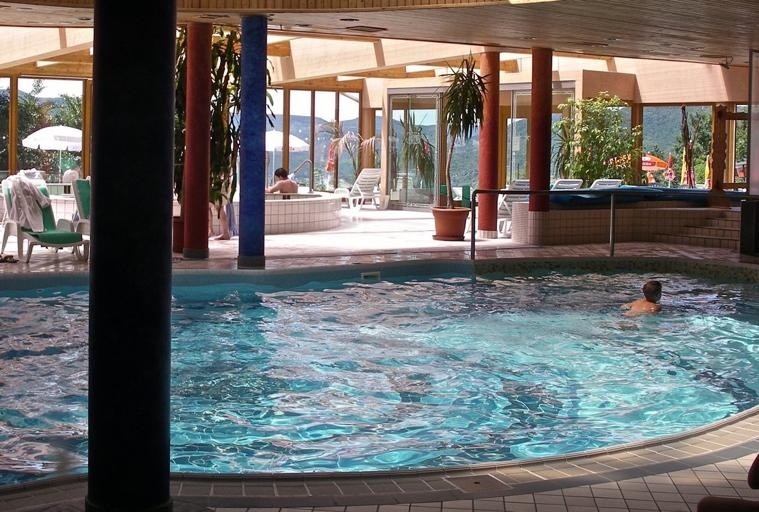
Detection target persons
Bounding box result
[698,453,759,512]
[619,280,663,318]
[208,188,230,241]
[62,163,80,184]
[265,169,298,199]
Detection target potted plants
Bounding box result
[172,24,277,253]
[428,48,497,241]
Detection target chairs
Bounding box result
[333,167,389,224]
[72,177,90,234]
[466,179,530,238]
[550,179,583,191]
[62,169,79,183]
[589,179,623,189]
[0,177,90,263]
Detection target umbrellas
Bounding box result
[604,151,667,171]
[22,124,82,183]
[663,152,675,188]
[326,141,337,189]
[265,128,310,185]
